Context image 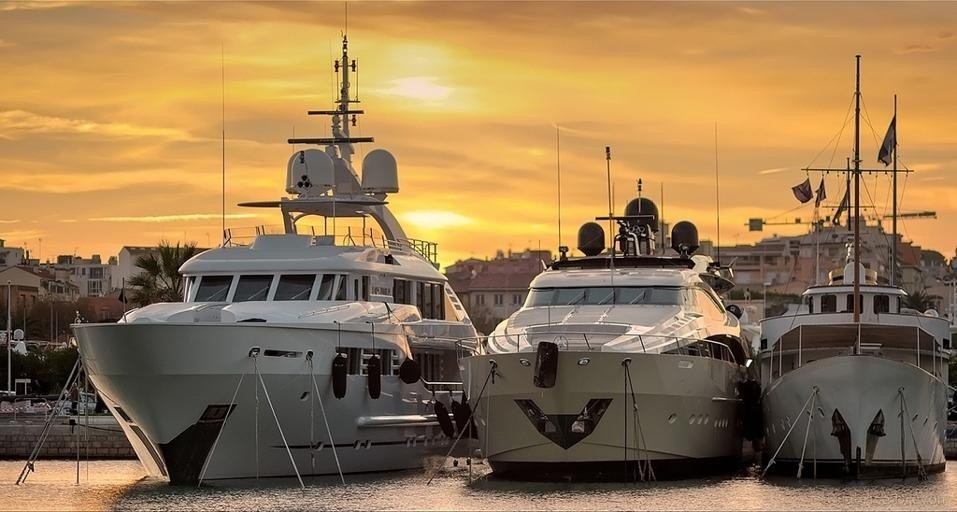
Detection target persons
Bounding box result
[67,382,82,416]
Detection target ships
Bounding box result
[68,0,486,488]
[745,54,956,480]
[454,143,757,480]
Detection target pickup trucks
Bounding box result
[59,392,108,414]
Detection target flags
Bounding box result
[877,113,897,169]
[815,177,827,209]
[790,177,814,203]
[831,185,852,225]
